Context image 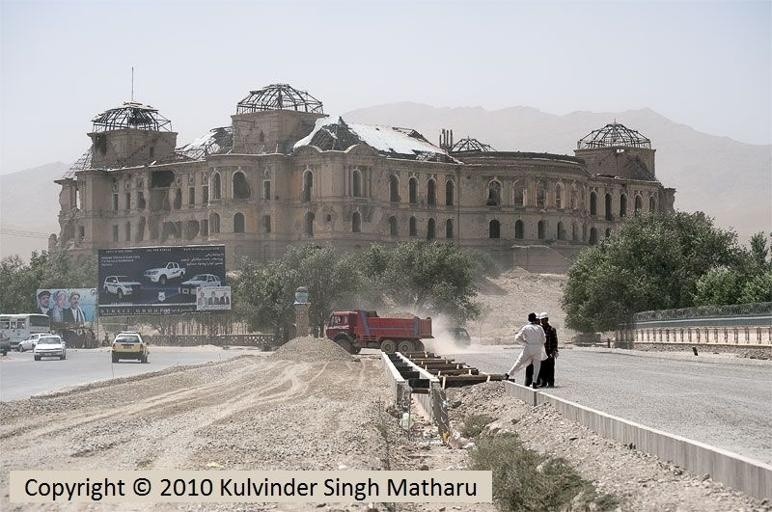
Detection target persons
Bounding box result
[208,291,219,305]
[523,312,541,386]
[505,312,549,389]
[198,291,207,305]
[538,312,558,387]
[37,290,52,314]
[220,292,229,304]
[66,291,86,321]
[50,290,68,322]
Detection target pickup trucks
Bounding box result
[142,262,186,286]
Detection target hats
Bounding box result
[38,291,80,302]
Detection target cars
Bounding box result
[177,274,222,296]
[112,331,149,363]
[0,329,12,356]
[32,334,67,360]
[18,332,53,352]
[103,276,144,300]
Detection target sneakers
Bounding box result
[501,373,509,380]
[525,382,554,388]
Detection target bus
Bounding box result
[0,313,51,349]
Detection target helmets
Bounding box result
[538,312,548,320]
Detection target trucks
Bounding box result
[326,309,434,354]
